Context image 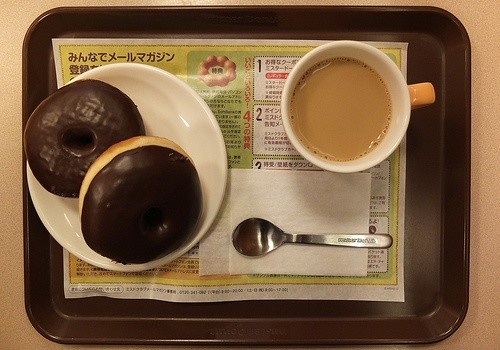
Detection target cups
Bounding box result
[280,40,435,174]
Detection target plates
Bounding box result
[26,62,229,272]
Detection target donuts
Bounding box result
[22,79,146,198]
[79,135,203,265]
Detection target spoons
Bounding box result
[231,218,392,257]
